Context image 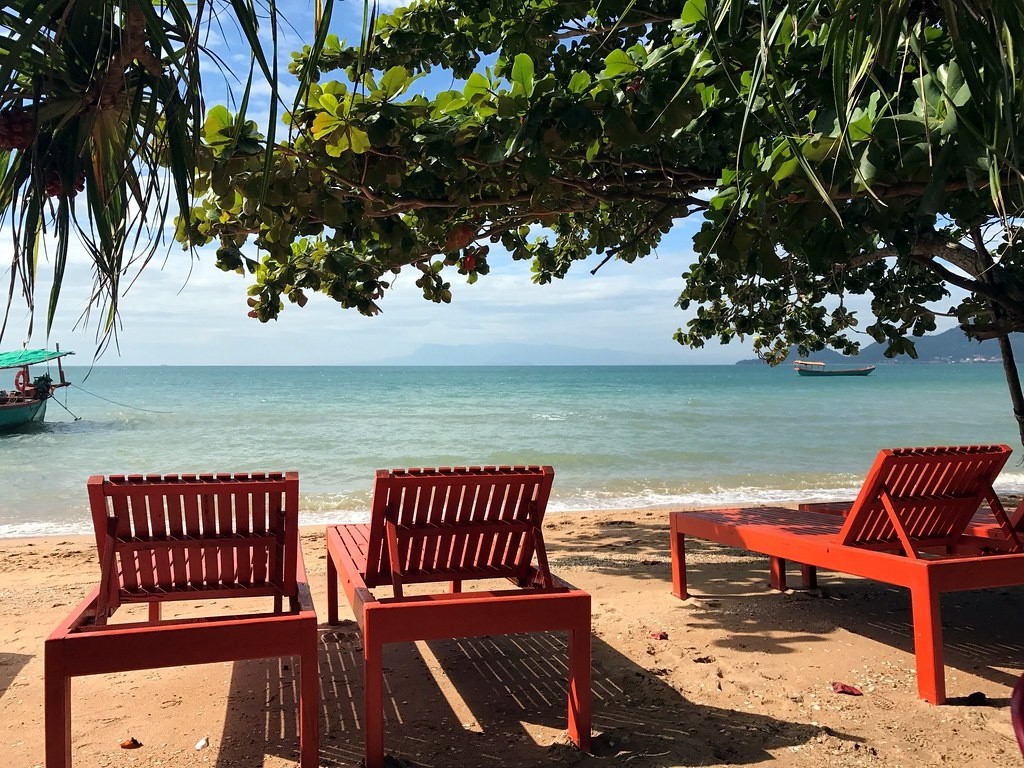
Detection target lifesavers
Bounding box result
[15,371,28,390]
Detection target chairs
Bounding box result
[798,498,1024,591]
[326,465,591,768]
[44,472,319,768]
[669,444,1024,704]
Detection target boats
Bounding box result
[794,359,877,375]
[0,343,76,425]
